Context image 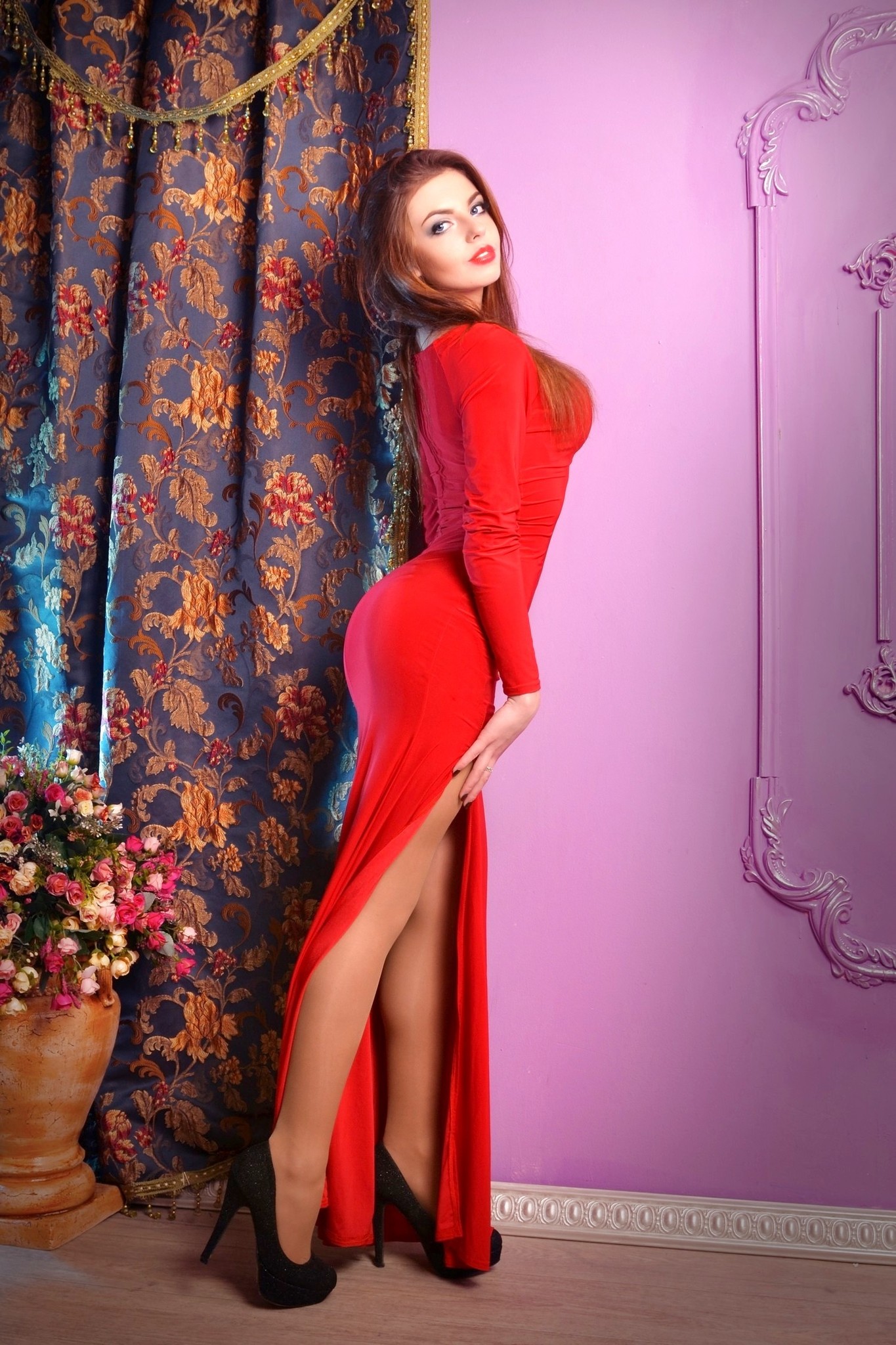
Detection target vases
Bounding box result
[0,990,122,1251]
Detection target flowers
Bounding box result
[0,731,198,1020]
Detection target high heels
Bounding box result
[200,1139,337,1306]
[373,1141,503,1279]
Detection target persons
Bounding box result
[195,150,595,1312]
